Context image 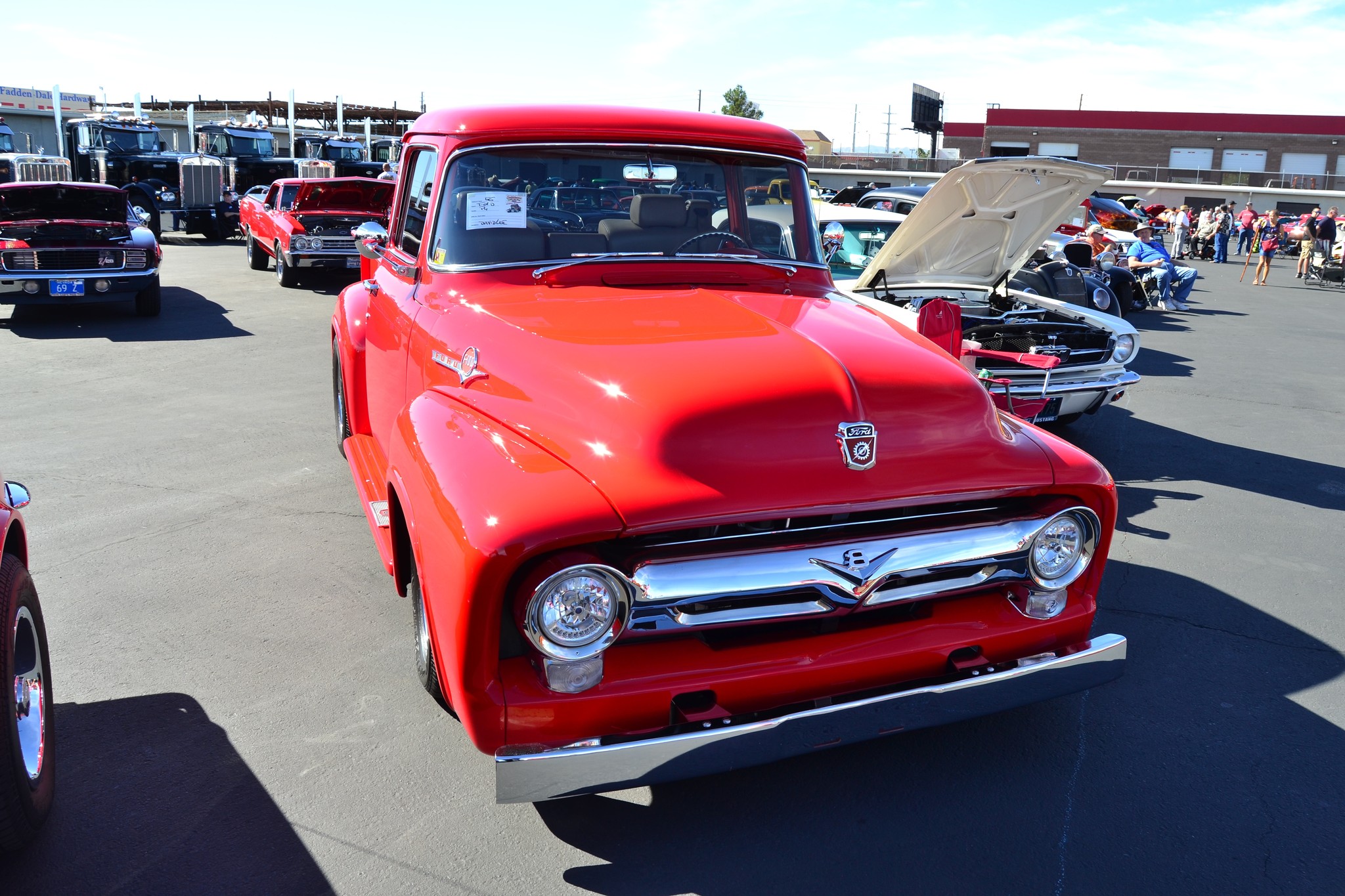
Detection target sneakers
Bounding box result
[1301,274,1315,279]
[1295,274,1302,278]
[1157,299,1176,311]
[1171,298,1189,310]
[1234,253,1241,255]
[1246,253,1250,256]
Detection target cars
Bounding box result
[811,157,1344,320]
[710,148,1140,431]
[238,175,399,287]
[0,474,55,827]
[0,180,163,320]
[332,110,1132,808]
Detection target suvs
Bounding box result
[57,113,224,242]
[191,118,337,240]
[290,132,400,181]
[364,140,405,164]
[1,121,73,182]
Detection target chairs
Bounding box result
[109,141,123,149]
[1274,231,1300,259]
[207,143,218,153]
[1228,226,1239,242]
[214,201,246,241]
[1127,242,1182,309]
[1304,241,1345,288]
[89,139,99,147]
[226,146,247,154]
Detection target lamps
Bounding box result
[1332,139,1338,145]
[1033,130,1038,136]
[1217,135,1222,141]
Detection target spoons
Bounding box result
[1113,247,1117,250]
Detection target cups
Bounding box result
[1112,249,1119,259]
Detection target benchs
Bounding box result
[453,191,719,264]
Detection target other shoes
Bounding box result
[1261,282,1266,285]
[1199,251,1203,255]
[1253,280,1258,285]
[1195,251,1199,254]
[1171,256,1215,263]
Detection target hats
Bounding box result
[1189,207,1195,210]
[1133,222,1156,238]
[1245,202,1252,206]
[1228,201,1237,205]
[1178,205,1190,211]
[1088,224,1108,234]
[1264,210,1269,213]
[1201,205,1206,208]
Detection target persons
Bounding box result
[523,178,539,202]
[1265,210,1269,215]
[1295,205,1338,281]
[1253,208,1284,285]
[570,176,599,208]
[1124,221,1198,311]
[639,177,720,205]
[1080,225,1146,312]
[1168,201,1260,264]
[1290,213,1298,220]
[487,174,500,190]
[377,163,398,182]
[554,182,569,209]
[869,182,879,190]
[215,189,241,230]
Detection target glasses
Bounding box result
[1313,211,1321,214]
[1332,211,1338,215]
